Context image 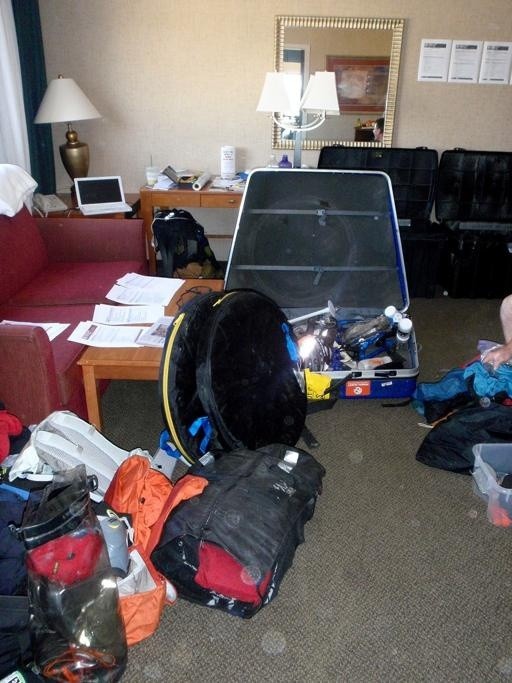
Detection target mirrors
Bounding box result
[271,16,405,151]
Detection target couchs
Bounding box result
[0,193,150,429]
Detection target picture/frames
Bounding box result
[320,51,390,115]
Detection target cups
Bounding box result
[145,165,161,185]
[220,146,237,178]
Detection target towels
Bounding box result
[0,163,39,216]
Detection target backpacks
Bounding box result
[8,411,155,503]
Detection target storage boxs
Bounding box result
[317,147,452,298]
[471,443,512,529]
[435,149,512,299]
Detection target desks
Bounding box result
[139,175,243,273]
[44,191,140,220]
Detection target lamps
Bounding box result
[260,64,338,170]
[34,70,103,180]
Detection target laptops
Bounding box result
[73,176,132,216]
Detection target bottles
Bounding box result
[267,152,293,169]
[384,305,411,356]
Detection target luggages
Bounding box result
[224,168,418,400]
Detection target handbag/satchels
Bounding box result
[152,209,224,280]
[149,441,325,619]
[158,287,307,465]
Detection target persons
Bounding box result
[482,293,512,370]
[373,118,384,142]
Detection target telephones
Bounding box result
[32,193,68,213]
